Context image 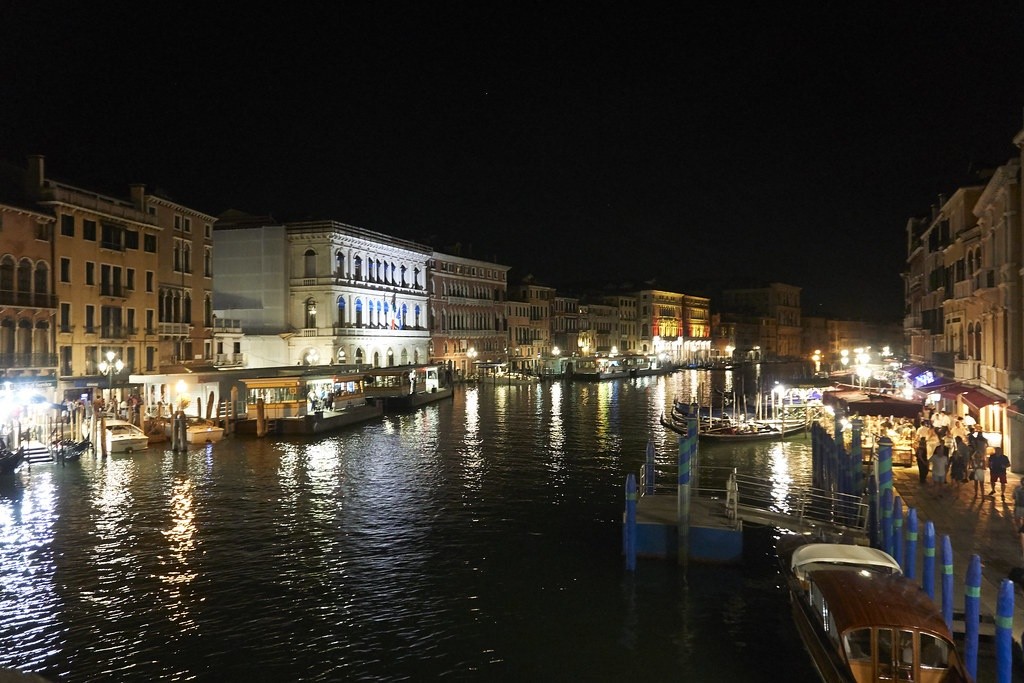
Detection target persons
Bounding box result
[306,383,335,411]
[871,397,988,502]
[93,392,136,414]
[915,438,928,481]
[990,448,1010,502]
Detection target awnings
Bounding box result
[941,383,974,401]
[917,377,956,394]
[962,388,1008,416]
[909,363,929,380]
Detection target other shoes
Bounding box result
[1001,495,1005,500]
[938,494,945,499]
[981,501,984,504]
[974,495,977,499]
[989,490,996,495]
[933,496,941,500]
[1017,528,1022,533]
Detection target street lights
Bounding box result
[552,346,560,359]
[307,349,320,397]
[466,347,478,375]
[99,350,124,404]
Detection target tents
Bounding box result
[823,387,924,468]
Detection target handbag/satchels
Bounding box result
[969,468,976,480]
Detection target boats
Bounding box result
[775,531,972,683]
[150,412,225,443]
[81,412,149,456]
[659,383,843,442]
[574,354,660,379]
